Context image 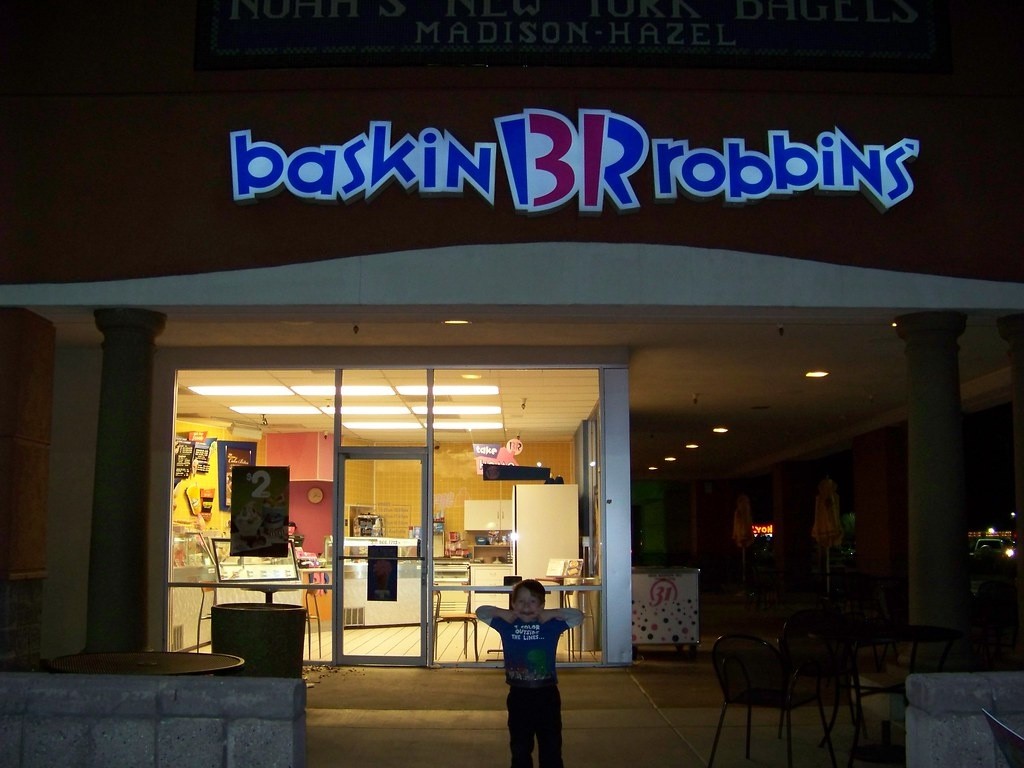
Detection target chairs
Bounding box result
[708,608,848,768]
[306,583,321,660]
[434,584,478,662]
[197,581,217,654]
[564,584,595,663]
[963,580,1019,671]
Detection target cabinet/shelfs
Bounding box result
[464,499,512,532]
[469,542,514,613]
[433,560,469,616]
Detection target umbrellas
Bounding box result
[732,495,754,583]
[810,475,844,593]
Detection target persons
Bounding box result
[475,579,585,768]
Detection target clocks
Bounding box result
[308,488,323,503]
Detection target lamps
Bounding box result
[705,482,712,492]
[228,420,263,439]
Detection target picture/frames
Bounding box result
[217,441,257,511]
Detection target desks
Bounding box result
[810,621,965,768]
[432,586,602,662]
[535,577,564,608]
[168,582,332,603]
[48,653,246,676]
